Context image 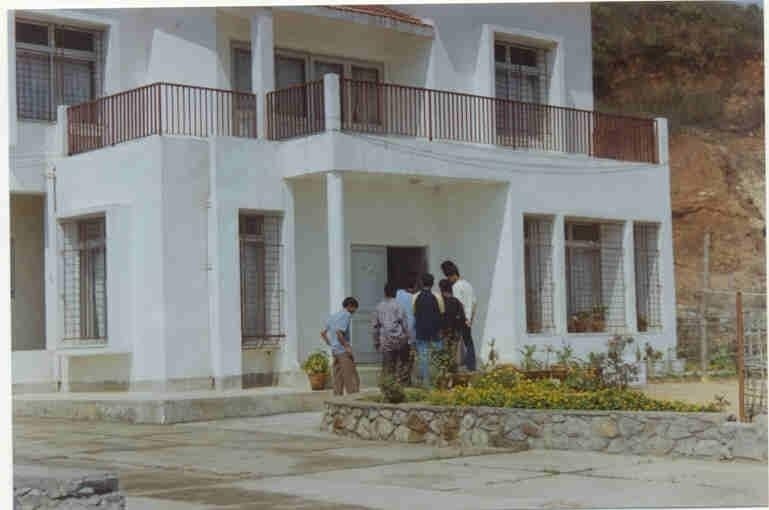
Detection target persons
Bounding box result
[370,258,479,389]
[318,294,362,397]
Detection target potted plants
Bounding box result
[519,345,572,382]
[570,304,609,332]
[429,350,456,392]
[302,351,328,390]
